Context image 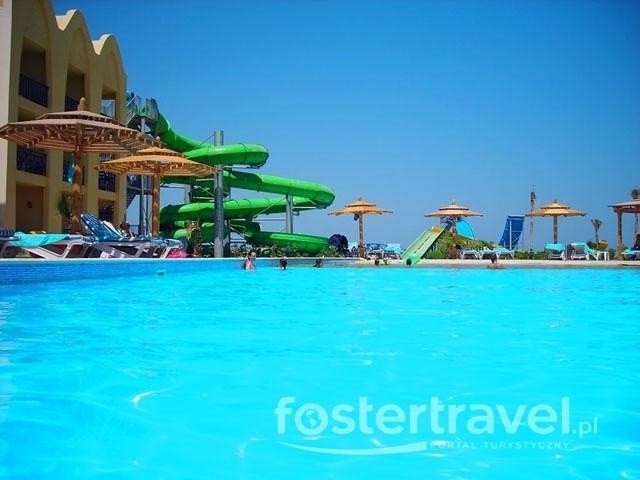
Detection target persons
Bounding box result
[405,257,413,267]
[356,245,368,255]
[313,258,324,268]
[487,254,506,270]
[277,258,288,271]
[374,257,388,266]
[244,251,258,270]
[235,247,261,256]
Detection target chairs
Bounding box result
[620,249,640,261]
[382,243,402,258]
[569,242,597,261]
[0,229,94,259]
[456,246,514,260]
[79,213,160,258]
[102,220,184,258]
[544,242,567,261]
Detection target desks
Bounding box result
[597,251,610,261]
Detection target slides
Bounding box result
[401,224,447,258]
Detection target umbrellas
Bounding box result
[526,200,587,248]
[2,97,168,239]
[423,200,487,257]
[327,197,396,254]
[93,133,222,238]
